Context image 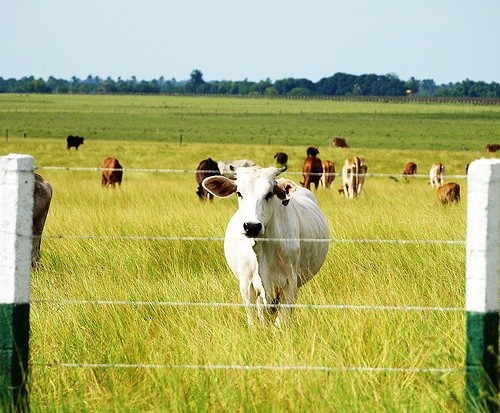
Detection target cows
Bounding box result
[102,157,122,188]
[403,162,418,175]
[320,161,337,188]
[200,165,329,329]
[486,144,500,153]
[307,146,320,158]
[433,182,461,204]
[337,157,368,198]
[429,162,444,187]
[300,157,323,191]
[196,159,219,203]
[217,160,255,175]
[67,134,85,151]
[32,174,52,265]
[331,138,350,148]
[274,152,288,165]
